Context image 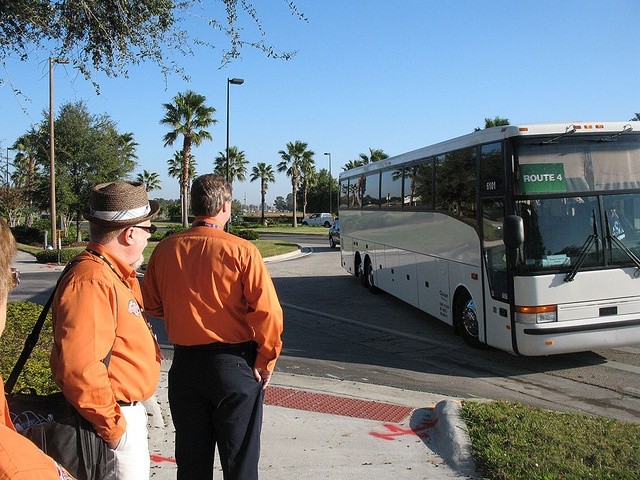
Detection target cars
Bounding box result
[329,220,340,248]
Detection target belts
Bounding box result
[116,400,138,406]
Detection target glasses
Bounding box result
[10,267,20,288]
[124,224,157,233]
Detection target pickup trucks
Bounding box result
[302,213,339,228]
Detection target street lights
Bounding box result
[50,58,69,249]
[226,78,244,232]
[324,153,332,213]
[7,148,15,183]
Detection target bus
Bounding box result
[339,121,640,356]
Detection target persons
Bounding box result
[50,181,163,480]
[0,218,76,480]
[140,174,284,480]
[605,201,635,241]
[263,218,270,227]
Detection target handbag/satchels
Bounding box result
[5,387,118,480]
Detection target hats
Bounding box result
[85,180,161,226]
